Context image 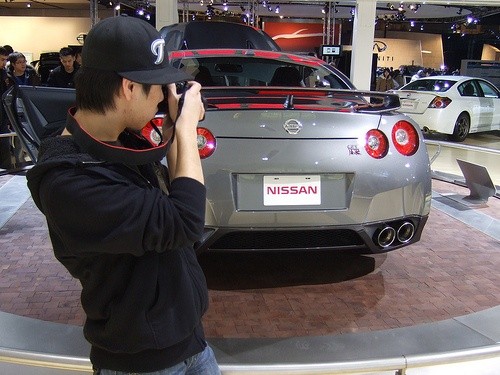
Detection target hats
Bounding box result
[81,13,196,85]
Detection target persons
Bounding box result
[376,66,462,92]
[27,16,221,375]
[0,45,80,168]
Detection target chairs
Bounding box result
[191,66,213,86]
[269,67,306,88]
[463,84,474,96]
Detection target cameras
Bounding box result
[155,80,207,122]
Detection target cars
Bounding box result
[2,48,430,273]
[387,74,500,141]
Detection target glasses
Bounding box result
[15,60,27,65]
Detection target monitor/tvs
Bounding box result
[319,45,342,56]
